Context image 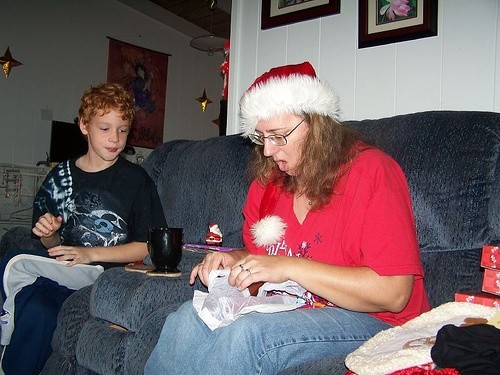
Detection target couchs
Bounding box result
[0,108,500,375]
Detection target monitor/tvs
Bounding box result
[49,120,89,162]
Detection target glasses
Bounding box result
[248,118,305,146]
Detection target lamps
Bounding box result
[190,0,228,56]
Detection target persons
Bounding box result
[206,224,223,247]
[0,83,168,375]
[143,60,431,375]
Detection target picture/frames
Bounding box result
[261,0,341,30]
[357,0,437,49]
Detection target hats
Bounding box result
[238,62,336,131]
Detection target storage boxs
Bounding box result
[480,244,500,270]
[454,291,500,307]
[481,269,500,296]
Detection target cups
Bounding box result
[147,227,184,273]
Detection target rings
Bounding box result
[246,269,251,274]
[239,264,244,271]
